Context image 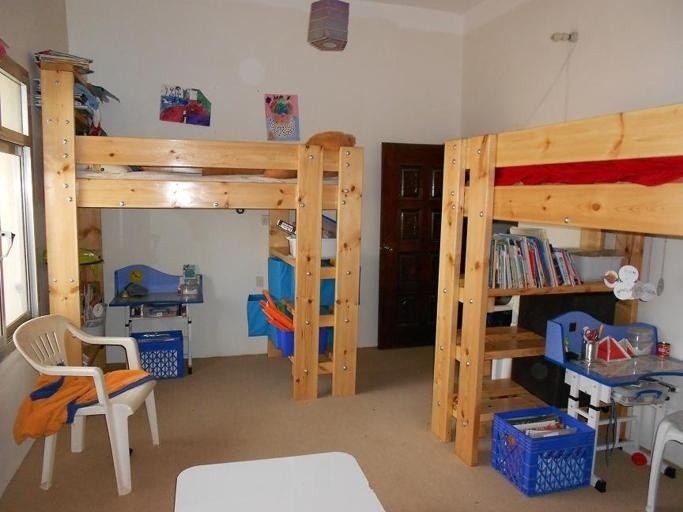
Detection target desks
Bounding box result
[174,451,387,512]
[111,264,204,375]
[544,310,683,493]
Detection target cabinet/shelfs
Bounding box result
[47,259,107,369]
[436,276,638,427]
[267,246,357,377]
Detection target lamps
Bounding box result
[550,31,579,43]
[308,1,349,50]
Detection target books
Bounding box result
[500,414,585,489]
[31,49,97,121]
[488,227,584,290]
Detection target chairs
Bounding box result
[646,410,683,511]
[14,314,160,495]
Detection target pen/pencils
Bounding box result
[585,323,604,342]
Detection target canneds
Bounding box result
[656,341,670,358]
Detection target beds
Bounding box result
[430,101,683,466]
[39,69,366,402]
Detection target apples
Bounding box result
[631,453,647,466]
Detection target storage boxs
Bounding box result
[571,251,625,284]
[247,236,336,358]
